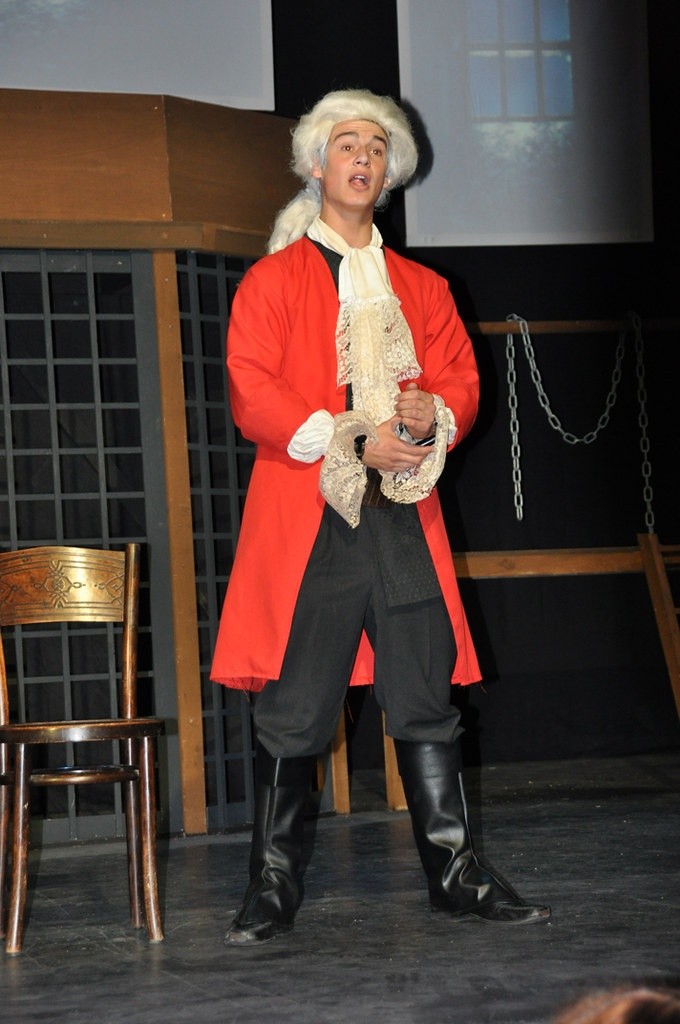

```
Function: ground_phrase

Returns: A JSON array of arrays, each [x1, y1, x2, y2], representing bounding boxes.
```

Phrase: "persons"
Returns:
[[210, 89, 555, 944]]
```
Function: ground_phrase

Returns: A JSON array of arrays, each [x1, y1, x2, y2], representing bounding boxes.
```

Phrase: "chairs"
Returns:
[[0, 540, 168, 958]]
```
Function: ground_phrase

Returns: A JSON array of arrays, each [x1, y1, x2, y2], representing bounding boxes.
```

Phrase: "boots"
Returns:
[[223, 735, 334, 945], [395, 733, 554, 926]]
